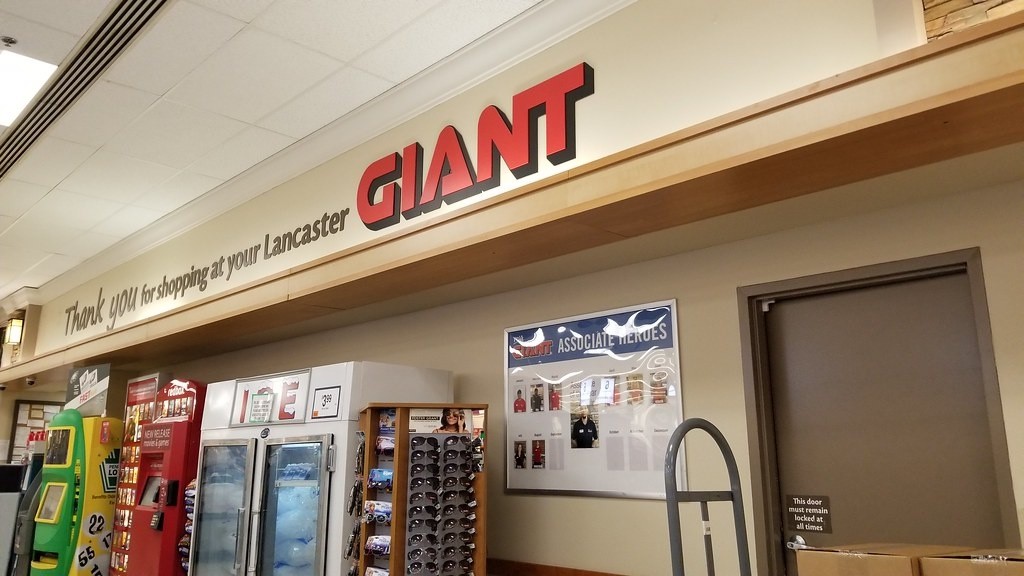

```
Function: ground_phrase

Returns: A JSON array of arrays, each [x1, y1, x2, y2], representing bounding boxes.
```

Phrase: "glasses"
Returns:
[[344, 433, 476, 576], [445, 411, 460, 417]]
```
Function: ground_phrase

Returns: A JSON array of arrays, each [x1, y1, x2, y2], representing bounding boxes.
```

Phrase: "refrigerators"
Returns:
[[187, 360, 454, 576]]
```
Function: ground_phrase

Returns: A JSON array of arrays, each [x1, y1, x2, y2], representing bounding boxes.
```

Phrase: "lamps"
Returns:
[[4, 317, 23, 345]]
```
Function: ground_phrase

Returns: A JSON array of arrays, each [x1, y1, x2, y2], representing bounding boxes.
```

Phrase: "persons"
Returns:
[[432, 409, 469, 433], [532, 441, 542, 468], [573, 405, 598, 448], [531, 388, 541, 412], [514, 443, 526, 468], [550, 384, 560, 410], [514, 389, 526, 412]]
